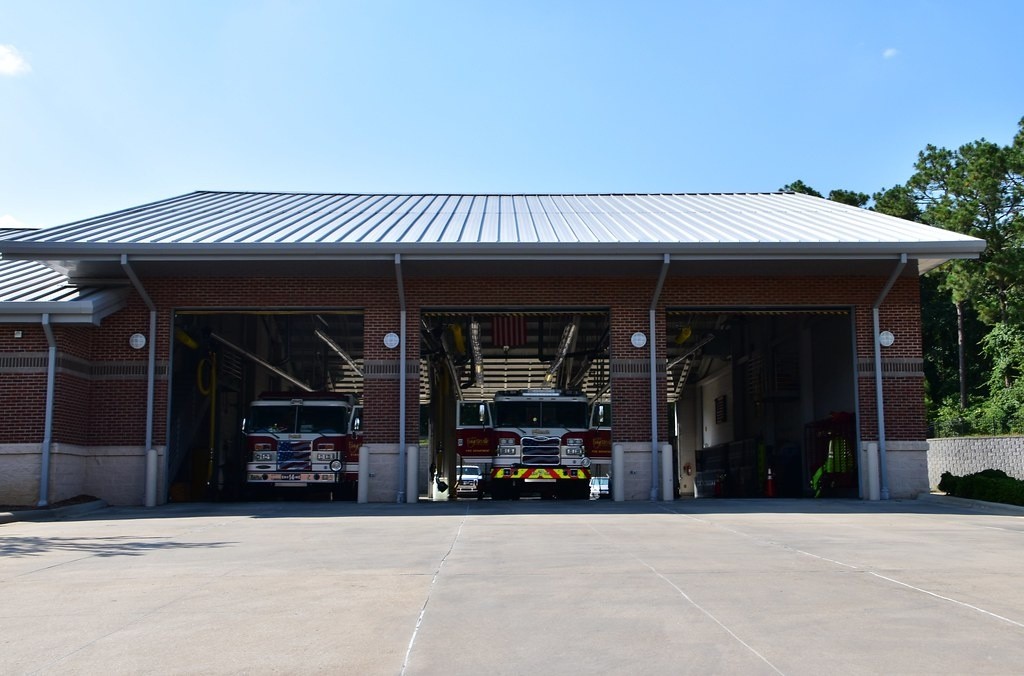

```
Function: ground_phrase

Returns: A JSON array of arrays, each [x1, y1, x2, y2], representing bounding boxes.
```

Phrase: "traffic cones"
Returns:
[[763, 467, 776, 498]]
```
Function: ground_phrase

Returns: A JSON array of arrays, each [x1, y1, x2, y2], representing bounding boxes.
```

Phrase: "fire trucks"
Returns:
[[239, 391, 362, 500], [455, 386, 611, 498]]
